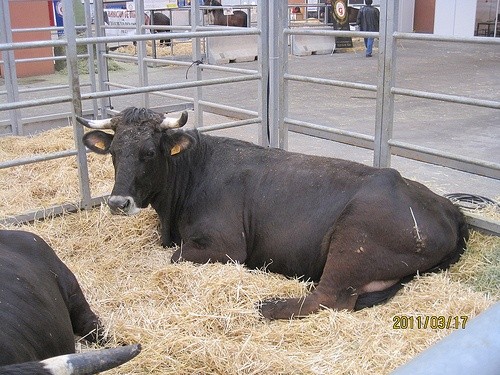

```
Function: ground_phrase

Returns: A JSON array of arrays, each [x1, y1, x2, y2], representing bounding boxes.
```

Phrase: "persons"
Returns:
[[356, 0, 380, 58]]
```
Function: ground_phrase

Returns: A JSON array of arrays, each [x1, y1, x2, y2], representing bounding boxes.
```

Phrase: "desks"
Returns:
[[477, 23, 500, 37]]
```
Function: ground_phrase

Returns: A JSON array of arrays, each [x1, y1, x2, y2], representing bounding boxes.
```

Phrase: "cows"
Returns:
[[0, 230, 141, 375], [74, 107, 469, 322]]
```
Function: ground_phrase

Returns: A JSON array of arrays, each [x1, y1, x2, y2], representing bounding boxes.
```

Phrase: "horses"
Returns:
[[149, 13, 170, 46], [203, 0, 247, 28]]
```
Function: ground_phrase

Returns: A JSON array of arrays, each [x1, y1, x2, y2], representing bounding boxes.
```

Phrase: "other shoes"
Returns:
[[366, 55, 373, 57]]
